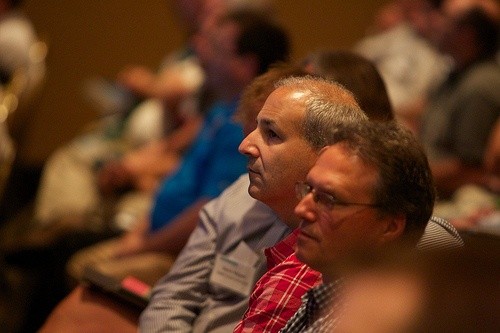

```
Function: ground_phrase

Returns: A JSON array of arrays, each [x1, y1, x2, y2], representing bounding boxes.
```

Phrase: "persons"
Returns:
[[2, 0, 499, 332]]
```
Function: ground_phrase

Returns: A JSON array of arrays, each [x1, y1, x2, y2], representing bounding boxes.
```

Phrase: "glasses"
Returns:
[[294, 181, 388, 210]]
